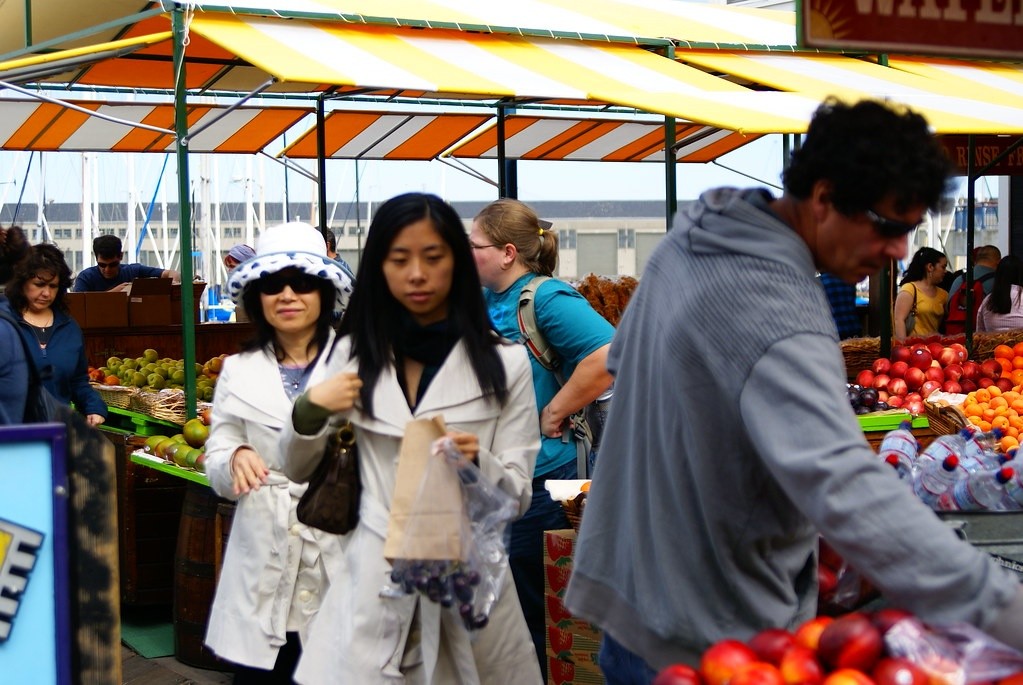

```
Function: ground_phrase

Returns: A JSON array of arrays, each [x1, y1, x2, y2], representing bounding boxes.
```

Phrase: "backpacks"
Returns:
[[944, 271, 996, 336]]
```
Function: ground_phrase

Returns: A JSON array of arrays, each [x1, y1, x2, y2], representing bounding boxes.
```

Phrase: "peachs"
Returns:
[[952, 384, 1023, 454], [653, 536, 1023, 685], [856, 340, 1013, 414]]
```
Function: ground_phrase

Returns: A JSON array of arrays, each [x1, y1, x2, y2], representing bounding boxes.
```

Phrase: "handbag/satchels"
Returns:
[[904, 282, 917, 337], [296, 423, 363, 536], [379, 441, 520, 630]]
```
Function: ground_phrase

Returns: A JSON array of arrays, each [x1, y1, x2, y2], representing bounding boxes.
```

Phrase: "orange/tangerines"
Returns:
[[993, 341, 1023, 385]]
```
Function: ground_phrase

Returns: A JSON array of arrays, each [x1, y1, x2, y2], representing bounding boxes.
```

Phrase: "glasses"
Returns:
[[225, 264, 235, 269], [253, 271, 327, 295], [861, 208, 922, 242], [97, 261, 120, 269]]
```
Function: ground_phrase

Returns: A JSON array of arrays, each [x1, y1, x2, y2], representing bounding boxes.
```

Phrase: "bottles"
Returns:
[[879, 419, 1023, 512]]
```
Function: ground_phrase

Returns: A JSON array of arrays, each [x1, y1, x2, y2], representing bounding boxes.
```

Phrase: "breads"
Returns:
[[576, 272, 639, 328]]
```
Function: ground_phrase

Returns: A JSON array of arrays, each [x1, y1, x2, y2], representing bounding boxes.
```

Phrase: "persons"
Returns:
[[468, 197, 622, 685], [569, 93, 1023, 685], [813, 245, 1023, 337], [224, 244, 257, 272], [314, 226, 354, 277], [0, 226, 108, 430], [74, 235, 201, 293], [202, 223, 358, 685], [275, 194, 549, 685]]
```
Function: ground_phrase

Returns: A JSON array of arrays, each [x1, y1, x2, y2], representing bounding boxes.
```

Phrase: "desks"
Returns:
[[95, 430, 216, 623]]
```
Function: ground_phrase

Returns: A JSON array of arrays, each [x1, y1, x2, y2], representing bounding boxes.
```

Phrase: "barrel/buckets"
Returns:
[[173, 487, 234, 673]]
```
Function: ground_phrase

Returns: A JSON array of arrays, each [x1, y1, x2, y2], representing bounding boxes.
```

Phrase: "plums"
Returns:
[[848, 384, 897, 415]]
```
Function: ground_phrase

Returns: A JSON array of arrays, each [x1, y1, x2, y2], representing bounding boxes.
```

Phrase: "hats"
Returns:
[[227, 220, 357, 321], [229, 244, 257, 262]]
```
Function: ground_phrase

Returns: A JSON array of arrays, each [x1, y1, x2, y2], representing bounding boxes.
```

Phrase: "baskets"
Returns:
[[839, 327, 1023, 455], [559, 498, 587, 536], [91, 384, 139, 410], [129, 391, 211, 427]]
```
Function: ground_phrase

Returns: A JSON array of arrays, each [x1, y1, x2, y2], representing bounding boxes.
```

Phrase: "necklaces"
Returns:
[[278, 361, 305, 389], [33, 308, 53, 332]]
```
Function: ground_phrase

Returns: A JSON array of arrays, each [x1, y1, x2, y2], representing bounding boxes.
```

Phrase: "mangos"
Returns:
[[142, 408, 213, 474]]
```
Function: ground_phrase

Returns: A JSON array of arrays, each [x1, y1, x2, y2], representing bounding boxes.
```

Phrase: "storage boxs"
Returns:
[[542, 528, 607, 685], [66, 277, 208, 327]]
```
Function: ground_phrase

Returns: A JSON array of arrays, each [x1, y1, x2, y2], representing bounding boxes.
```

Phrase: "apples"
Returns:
[[88, 348, 231, 402]]
